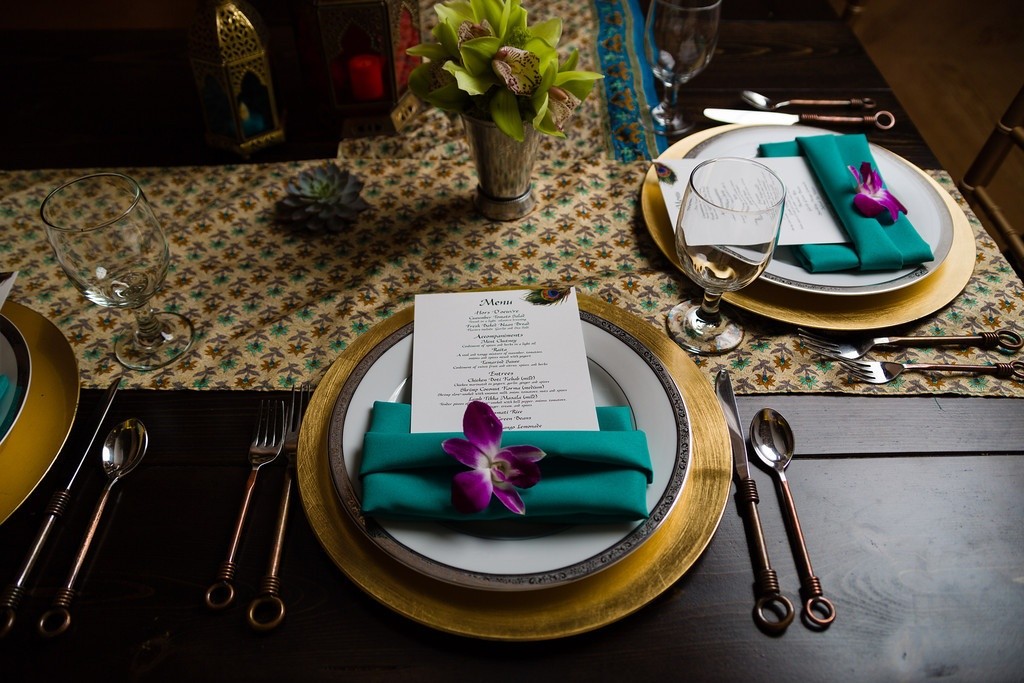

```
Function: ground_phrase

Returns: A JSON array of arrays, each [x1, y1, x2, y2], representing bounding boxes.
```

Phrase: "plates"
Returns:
[[296, 283, 734, 642], [641, 124, 976, 330], [0, 300, 81, 526]]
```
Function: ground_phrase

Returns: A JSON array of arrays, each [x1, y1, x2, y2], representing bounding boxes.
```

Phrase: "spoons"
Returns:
[[38, 418, 150, 637], [738, 89, 878, 112], [749, 407, 836, 627]]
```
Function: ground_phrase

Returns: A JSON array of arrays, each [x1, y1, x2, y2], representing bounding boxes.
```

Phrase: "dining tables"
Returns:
[[0, 0, 1024, 683]]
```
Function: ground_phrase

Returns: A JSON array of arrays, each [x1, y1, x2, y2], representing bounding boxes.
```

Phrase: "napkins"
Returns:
[[759, 134, 935, 274], [360, 401, 654, 521]]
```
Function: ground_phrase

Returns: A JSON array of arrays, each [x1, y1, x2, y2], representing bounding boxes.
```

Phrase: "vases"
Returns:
[[461, 111, 541, 220]]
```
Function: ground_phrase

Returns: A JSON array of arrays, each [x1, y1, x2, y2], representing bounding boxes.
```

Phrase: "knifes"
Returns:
[[0, 375, 121, 636], [703, 108, 897, 129], [715, 367, 794, 631]]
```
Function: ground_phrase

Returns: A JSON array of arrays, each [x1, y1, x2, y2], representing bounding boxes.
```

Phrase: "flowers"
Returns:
[[847, 162, 908, 221], [443, 401, 547, 516], [407, 0, 605, 141]]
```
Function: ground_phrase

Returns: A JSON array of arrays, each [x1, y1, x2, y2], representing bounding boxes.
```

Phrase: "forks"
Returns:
[[206, 382, 311, 629], [794, 324, 1024, 384]]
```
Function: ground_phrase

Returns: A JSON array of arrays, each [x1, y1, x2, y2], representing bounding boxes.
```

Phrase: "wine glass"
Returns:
[[38, 172, 195, 372], [637, 0, 721, 134], [662, 157, 788, 355]]
